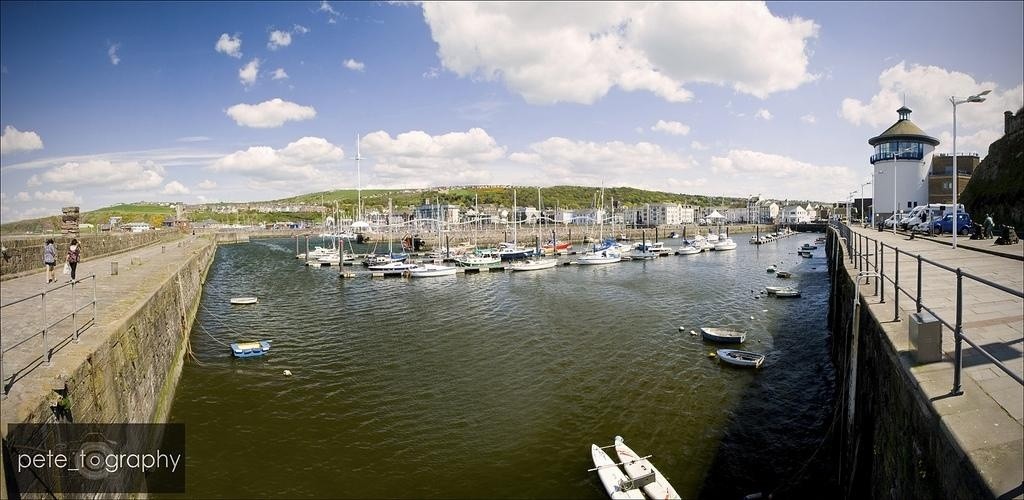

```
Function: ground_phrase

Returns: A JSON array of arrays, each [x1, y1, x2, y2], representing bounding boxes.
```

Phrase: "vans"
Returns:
[[884, 203, 972, 235]]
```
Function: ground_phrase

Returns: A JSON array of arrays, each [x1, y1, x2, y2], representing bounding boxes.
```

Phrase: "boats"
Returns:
[[798, 237, 826, 258], [700, 327, 747, 344], [776, 272, 791, 278], [766, 266, 777, 272], [613, 435, 681, 499], [765, 286, 802, 294], [716, 348, 765, 369], [749, 227, 792, 244], [587, 436, 646, 500], [776, 290, 798, 296], [229, 297, 258, 305], [230, 342, 270, 358]]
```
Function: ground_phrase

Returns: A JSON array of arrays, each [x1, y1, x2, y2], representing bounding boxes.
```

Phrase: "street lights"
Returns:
[[846, 190, 857, 227], [872, 170, 888, 230], [892, 146, 918, 234], [946, 89, 991, 249], [860, 181, 872, 229]]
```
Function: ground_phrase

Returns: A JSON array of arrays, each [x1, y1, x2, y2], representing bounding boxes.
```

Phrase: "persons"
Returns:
[[67, 239, 81, 283], [983, 213, 995, 239], [44, 238, 57, 284]]
